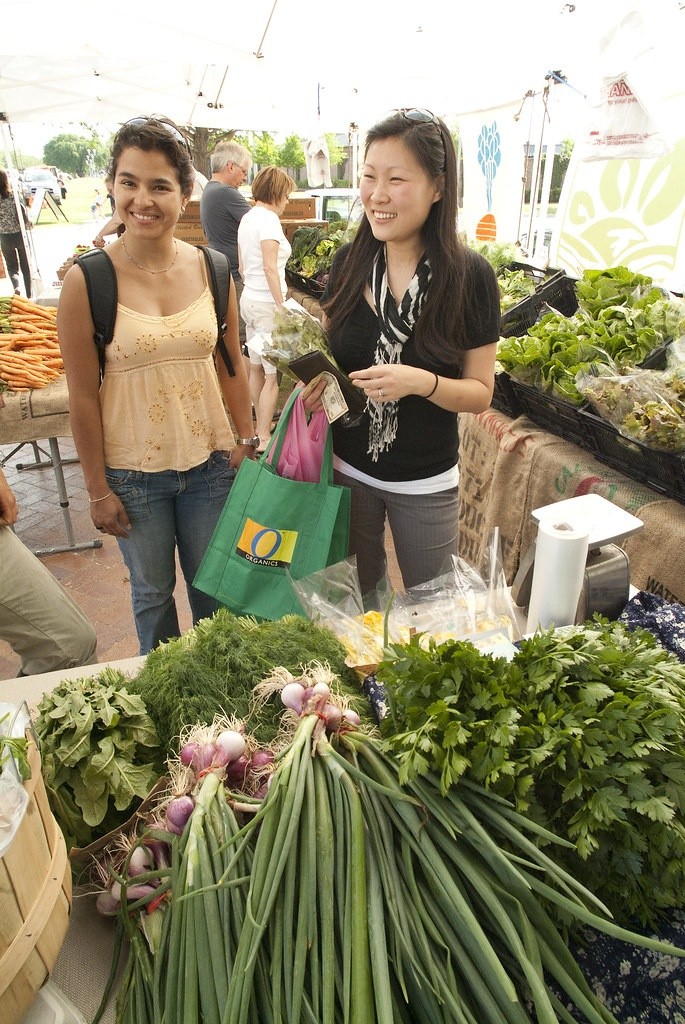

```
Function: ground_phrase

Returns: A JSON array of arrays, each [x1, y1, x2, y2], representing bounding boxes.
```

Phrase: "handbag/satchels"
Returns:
[[192, 387, 351, 623], [0, 251, 6, 278]]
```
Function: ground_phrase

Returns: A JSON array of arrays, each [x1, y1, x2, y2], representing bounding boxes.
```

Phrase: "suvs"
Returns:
[[21, 169, 62, 205]]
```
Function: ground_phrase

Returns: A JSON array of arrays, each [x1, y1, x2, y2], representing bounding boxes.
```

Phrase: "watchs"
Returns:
[[236, 437, 260, 448]]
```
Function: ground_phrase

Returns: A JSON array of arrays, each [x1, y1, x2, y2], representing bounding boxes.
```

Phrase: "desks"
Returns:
[[288, 283, 685, 612], [0, 369, 101, 557], [0, 572, 644, 1023]]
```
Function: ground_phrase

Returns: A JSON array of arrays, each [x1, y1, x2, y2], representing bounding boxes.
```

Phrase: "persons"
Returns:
[[198, 141, 282, 422], [0, 463, 102, 678], [236, 164, 291, 457], [0, 172, 35, 300], [57, 115, 260, 658], [293, 107, 502, 616]]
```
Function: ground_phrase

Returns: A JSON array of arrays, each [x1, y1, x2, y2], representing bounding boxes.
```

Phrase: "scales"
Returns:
[[511, 493, 644, 625]]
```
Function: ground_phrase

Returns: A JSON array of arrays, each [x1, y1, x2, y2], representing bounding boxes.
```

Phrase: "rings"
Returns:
[[378, 389, 383, 396]]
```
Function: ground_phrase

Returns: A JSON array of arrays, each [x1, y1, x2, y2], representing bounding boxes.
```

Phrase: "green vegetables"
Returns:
[[259, 316, 341, 383], [291, 219, 361, 276], [466, 240, 685, 455], [0, 607, 356, 872], [373, 611, 685, 931]]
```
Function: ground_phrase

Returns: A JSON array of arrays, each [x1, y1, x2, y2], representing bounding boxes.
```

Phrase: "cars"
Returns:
[[44, 165, 73, 182]]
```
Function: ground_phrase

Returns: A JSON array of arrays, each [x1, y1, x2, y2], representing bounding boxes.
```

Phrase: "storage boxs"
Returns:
[[284, 246, 685, 507], [173, 197, 329, 252]]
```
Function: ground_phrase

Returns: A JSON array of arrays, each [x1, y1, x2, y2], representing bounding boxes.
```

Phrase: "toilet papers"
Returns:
[[525, 514, 590, 638]]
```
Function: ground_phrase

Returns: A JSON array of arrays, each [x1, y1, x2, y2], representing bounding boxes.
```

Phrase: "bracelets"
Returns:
[[89, 490, 114, 503], [423, 370, 438, 398]]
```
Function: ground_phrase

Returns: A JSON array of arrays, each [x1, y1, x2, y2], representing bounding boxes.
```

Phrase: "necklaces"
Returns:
[[120, 232, 179, 274]]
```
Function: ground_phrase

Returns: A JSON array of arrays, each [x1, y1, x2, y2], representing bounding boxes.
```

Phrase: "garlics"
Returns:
[[96, 684, 361, 915]]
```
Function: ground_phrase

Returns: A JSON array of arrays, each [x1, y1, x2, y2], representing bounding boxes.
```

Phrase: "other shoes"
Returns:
[[15, 289, 21, 296]]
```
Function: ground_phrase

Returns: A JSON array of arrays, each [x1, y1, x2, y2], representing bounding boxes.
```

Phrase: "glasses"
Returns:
[[284, 192, 289, 200], [227, 161, 248, 176], [376, 107, 441, 134], [117, 118, 187, 154]]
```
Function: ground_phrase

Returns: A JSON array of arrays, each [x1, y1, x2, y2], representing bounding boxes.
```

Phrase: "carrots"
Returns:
[[0, 295, 65, 391]]
